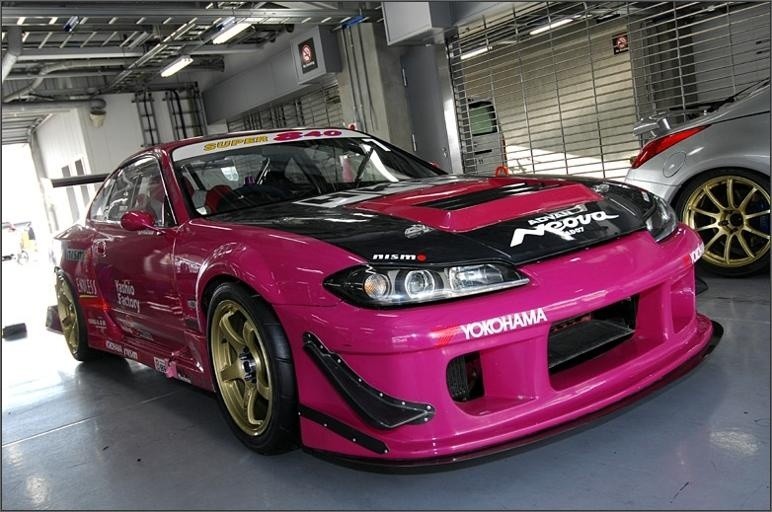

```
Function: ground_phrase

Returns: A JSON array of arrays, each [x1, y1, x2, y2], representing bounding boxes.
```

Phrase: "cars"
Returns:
[[2, 223, 37, 263]]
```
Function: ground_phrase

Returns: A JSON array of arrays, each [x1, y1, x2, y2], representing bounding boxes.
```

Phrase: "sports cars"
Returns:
[[619, 73, 771, 275], [42, 122, 729, 471]]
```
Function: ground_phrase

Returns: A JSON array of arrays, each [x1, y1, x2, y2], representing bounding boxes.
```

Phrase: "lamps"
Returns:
[[159, 54, 194, 77]]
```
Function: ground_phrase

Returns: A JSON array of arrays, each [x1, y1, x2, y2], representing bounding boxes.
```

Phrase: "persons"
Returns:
[[19, 227, 32, 251], [26, 222, 39, 263]]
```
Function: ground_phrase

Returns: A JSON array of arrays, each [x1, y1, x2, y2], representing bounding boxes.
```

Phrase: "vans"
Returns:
[[468, 100, 506, 176]]
